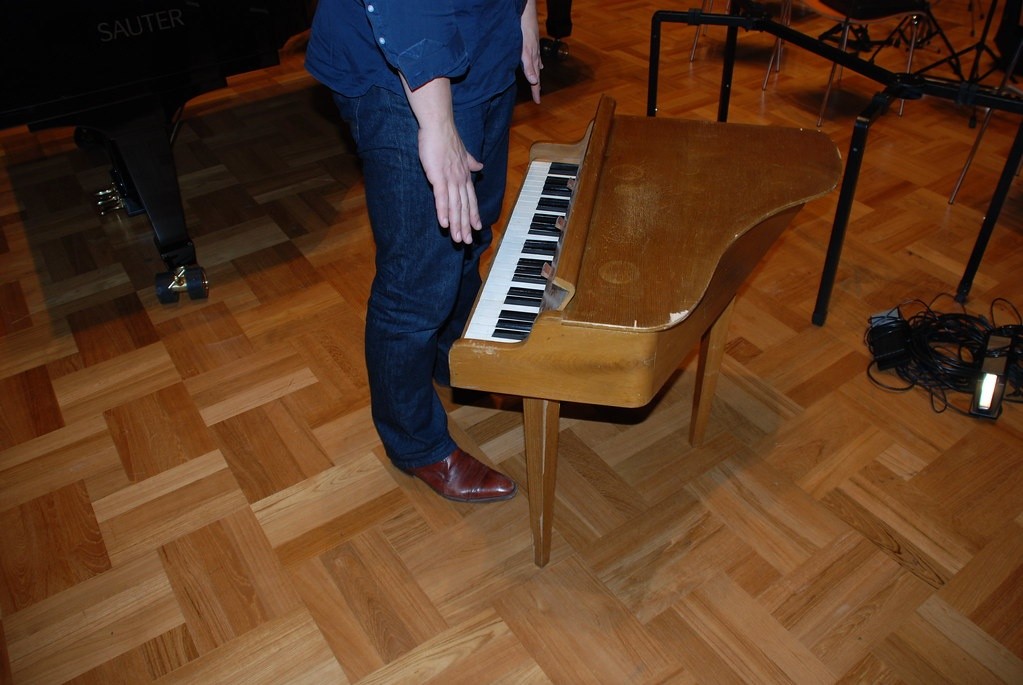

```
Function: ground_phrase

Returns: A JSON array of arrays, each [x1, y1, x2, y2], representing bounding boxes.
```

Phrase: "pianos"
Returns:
[[448, 94, 844, 569]]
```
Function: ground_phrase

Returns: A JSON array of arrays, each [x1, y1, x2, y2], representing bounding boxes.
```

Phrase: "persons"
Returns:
[[304, 0, 545, 502]]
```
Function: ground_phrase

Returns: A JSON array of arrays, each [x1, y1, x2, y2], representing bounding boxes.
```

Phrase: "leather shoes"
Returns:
[[391, 446, 518, 503]]
